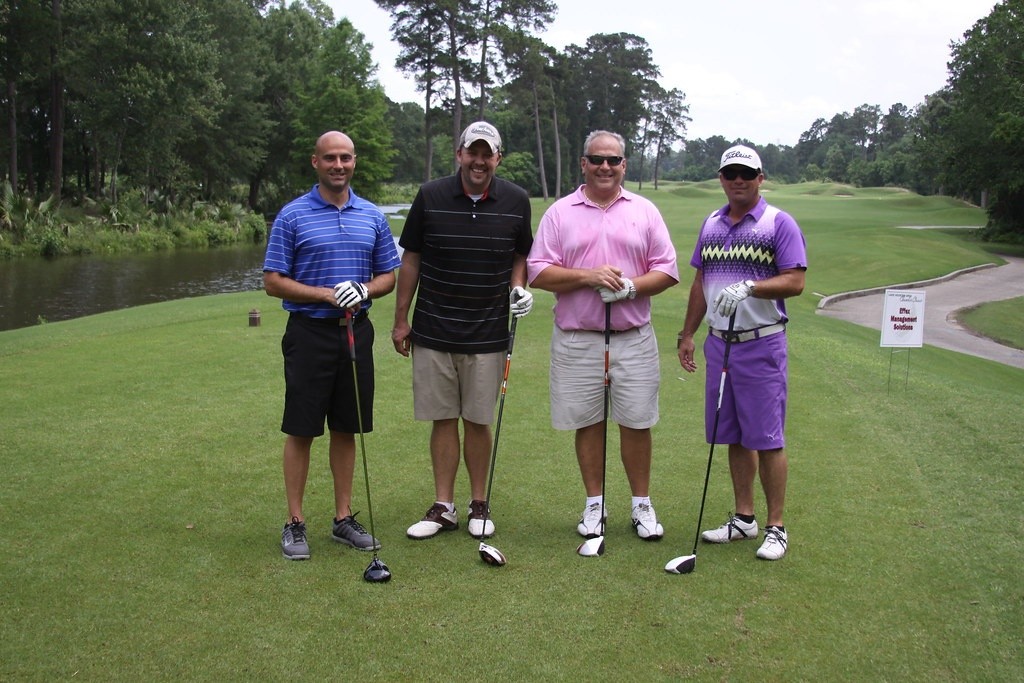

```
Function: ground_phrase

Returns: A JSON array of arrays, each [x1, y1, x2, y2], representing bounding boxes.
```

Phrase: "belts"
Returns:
[[296, 311, 367, 326], [589, 327, 635, 334], [709, 323, 785, 342]]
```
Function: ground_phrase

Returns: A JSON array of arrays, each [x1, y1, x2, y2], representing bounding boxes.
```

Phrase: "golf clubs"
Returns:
[[575, 302, 613, 558], [477, 293, 523, 567], [345, 308, 392, 584], [664, 305, 740, 576]]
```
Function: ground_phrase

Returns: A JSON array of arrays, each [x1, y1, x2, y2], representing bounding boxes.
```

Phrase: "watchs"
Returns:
[[745, 281, 755, 293], [628, 280, 636, 300]]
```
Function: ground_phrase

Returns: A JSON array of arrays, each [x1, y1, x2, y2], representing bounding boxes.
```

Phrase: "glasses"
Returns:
[[585, 155, 623, 166], [720, 168, 758, 181]]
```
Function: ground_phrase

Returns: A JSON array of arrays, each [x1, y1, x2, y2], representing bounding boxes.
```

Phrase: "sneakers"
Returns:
[[756, 525, 787, 560], [577, 502, 608, 536], [406, 501, 458, 538], [282, 522, 311, 560], [332, 517, 382, 551], [631, 502, 664, 540], [702, 517, 758, 542], [468, 501, 495, 537]]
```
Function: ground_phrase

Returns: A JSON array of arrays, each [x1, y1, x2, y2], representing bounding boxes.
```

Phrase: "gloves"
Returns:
[[713, 283, 751, 317], [334, 280, 368, 308], [593, 278, 636, 302], [510, 287, 533, 317]]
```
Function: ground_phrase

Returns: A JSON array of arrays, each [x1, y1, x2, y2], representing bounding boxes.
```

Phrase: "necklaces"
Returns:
[[583, 189, 620, 208]]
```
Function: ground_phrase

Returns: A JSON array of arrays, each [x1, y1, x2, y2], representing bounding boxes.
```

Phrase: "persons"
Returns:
[[392, 121, 534, 540], [526, 130, 679, 539], [263, 130, 400, 560], [676, 144, 807, 559]]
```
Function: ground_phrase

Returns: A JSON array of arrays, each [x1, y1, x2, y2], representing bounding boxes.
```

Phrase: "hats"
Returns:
[[459, 121, 501, 153], [717, 146, 762, 172]]
